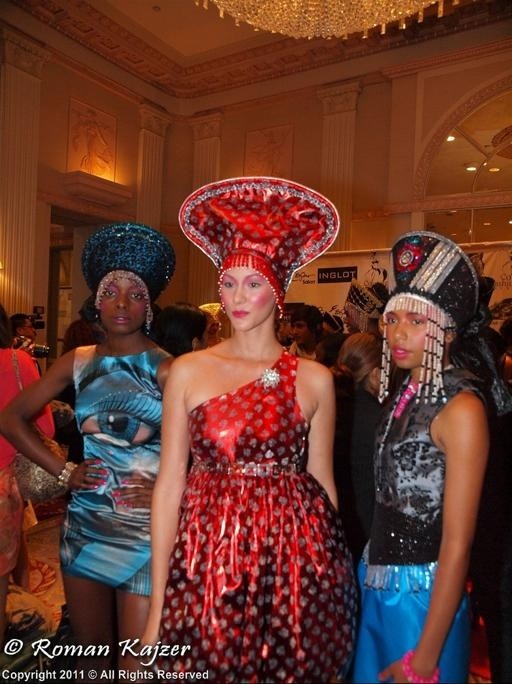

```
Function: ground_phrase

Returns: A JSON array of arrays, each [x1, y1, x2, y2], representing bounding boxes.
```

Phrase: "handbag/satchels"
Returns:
[[12, 439, 68, 500]]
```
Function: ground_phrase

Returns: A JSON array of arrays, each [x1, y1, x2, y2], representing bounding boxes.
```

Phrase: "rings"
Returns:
[[23, 343, 28, 347], [31, 350, 35, 355]]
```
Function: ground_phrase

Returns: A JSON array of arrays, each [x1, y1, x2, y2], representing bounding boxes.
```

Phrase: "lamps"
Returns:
[[189, 0, 460, 41]]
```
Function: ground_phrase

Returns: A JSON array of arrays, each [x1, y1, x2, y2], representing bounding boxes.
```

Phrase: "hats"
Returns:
[[378, 230, 479, 403], [81, 223, 176, 330], [178, 176, 340, 320], [343, 278, 388, 330]]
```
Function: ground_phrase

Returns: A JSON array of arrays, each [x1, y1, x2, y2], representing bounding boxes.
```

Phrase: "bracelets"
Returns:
[[402, 651, 441, 683]]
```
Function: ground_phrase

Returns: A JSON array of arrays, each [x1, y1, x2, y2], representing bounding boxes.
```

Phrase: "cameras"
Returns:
[[15, 335, 50, 358]]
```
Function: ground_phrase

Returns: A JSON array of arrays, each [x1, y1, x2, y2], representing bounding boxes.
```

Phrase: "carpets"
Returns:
[[7, 494, 72, 633]]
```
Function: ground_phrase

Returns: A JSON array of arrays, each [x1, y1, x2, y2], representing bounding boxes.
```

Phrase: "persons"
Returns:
[[56, 292, 511, 575], [10, 312, 37, 343], [355, 293, 491, 683], [139, 252, 360, 683], [1, 270, 179, 682], [0, 305, 56, 650]]
